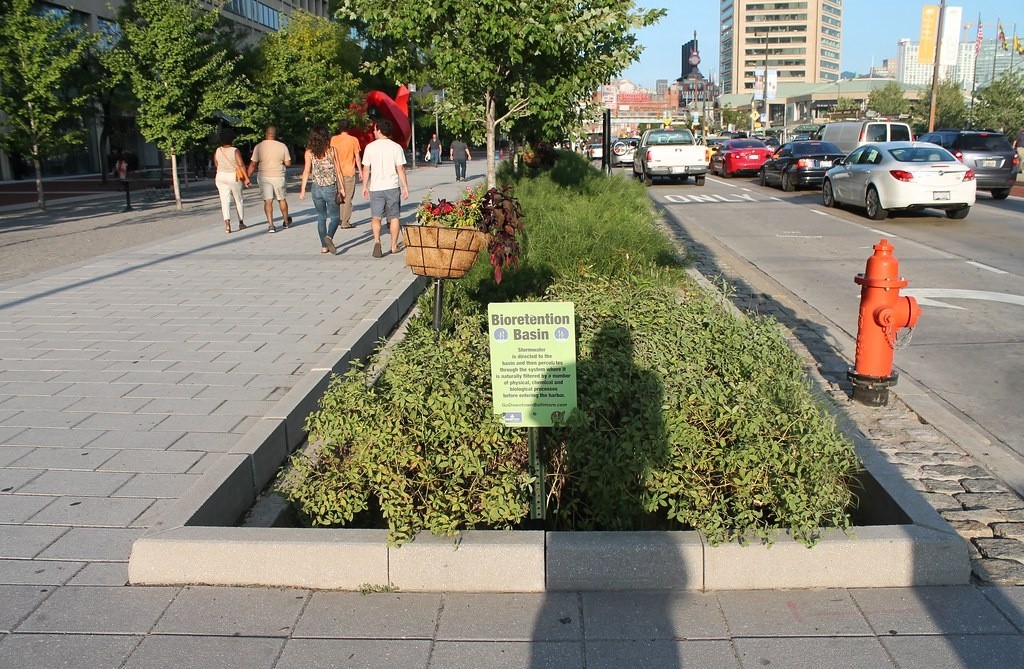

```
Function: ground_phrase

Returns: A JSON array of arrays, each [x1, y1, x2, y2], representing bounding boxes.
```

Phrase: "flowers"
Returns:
[[415, 185, 525, 284]]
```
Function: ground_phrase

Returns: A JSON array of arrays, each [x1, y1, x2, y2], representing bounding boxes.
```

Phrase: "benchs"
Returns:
[[896, 153, 941, 162]]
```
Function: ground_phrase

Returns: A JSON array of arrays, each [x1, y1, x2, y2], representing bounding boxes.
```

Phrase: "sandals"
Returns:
[[341, 225, 354, 229]]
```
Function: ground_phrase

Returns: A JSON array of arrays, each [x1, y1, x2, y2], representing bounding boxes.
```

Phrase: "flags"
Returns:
[[974, 20, 983, 56], [1014, 33, 1024, 59], [998, 24, 1009, 50]]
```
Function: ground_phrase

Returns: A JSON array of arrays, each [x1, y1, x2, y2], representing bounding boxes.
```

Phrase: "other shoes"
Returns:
[[268, 227, 276, 232], [226, 226, 231, 233], [282, 217, 293, 228], [324, 236, 336, 254], [457, 178, 464, 182], [239, 224, 245, 229], [322, 248, 328, 253]]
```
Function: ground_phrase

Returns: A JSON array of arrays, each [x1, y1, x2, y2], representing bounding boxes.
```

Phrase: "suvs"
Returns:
[[915, 129, 1019, 199]]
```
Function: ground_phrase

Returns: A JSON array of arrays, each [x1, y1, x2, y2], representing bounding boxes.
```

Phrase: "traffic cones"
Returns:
[[500, 149, 505, 158]]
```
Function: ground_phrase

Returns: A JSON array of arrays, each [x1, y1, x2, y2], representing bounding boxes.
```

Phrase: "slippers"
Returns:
[[373, 244, 382, 258], [393, 246, 403, 254]]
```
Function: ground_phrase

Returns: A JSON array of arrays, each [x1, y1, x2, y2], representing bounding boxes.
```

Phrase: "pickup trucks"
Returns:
[[631, 128, 709, 187]]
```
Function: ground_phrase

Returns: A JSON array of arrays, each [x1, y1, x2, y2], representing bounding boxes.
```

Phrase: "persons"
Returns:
[[246, 125, 292, 232], [330, 119, 363, 229], [300, 122, 346, 254], [361, 121, 409, 258], [214, 129, 251, 234], [450, 132, 472, 182], [427, 133, 442, 167], [565, 139, 592, 161]]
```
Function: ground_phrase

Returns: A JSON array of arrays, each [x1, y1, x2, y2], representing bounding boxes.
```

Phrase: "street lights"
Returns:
[[408, 83, 416, 166], [433, 94, 441, 163]]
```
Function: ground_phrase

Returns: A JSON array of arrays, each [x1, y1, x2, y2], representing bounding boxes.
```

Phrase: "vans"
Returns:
[[808, 120, 915, 162]]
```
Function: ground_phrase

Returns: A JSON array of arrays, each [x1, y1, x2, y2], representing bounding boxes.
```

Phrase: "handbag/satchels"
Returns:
[[235, 170, 245, 180], [336, 192, 345, 205], [425, 150, 431, 161]]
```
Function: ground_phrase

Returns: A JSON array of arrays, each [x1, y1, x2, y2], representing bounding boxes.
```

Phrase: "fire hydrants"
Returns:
[[847, 237, 923, 407]]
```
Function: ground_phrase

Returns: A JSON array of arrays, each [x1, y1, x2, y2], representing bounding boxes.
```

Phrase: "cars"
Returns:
[[788, 124, 814, 142], [710, 139, 773, 178], [760, 142, 848, 192], [755, 136, 781, 151], [697, 137, 732, 163], [823, 141, 977, 220], [587, 144, 603, 160], [718, 131, 747, 138], [610, 137, 641, 167]]
